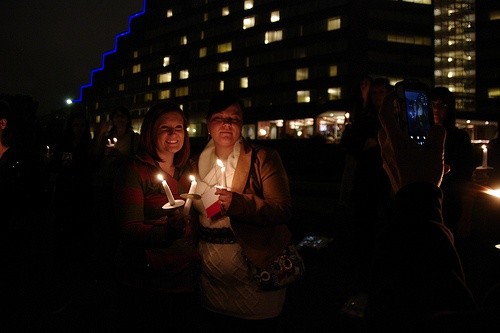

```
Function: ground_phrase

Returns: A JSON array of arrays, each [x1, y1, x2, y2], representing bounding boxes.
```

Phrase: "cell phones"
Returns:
[[403, 80, 436, 148]]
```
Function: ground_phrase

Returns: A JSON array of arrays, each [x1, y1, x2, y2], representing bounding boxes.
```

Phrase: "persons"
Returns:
[[278, 86, 499, 333], [0, 94, 145, 333], [116, 102, 201, 333], [191, 98, 292, 333]]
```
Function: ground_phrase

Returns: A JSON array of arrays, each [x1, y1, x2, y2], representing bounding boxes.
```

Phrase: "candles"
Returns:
[[215, 158, 226, 189], [108, 139, 111, 145], [156, 173, 175, 206], [481, 144, 488, 167], [183, 174, 198, 216], [46, 145, 50, 158], [113, 137, 118, 144]]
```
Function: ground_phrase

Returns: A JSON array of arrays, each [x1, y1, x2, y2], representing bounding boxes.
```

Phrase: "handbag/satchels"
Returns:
[[252, 249, 305, 292]]
